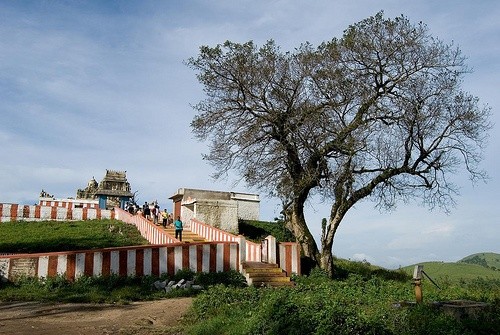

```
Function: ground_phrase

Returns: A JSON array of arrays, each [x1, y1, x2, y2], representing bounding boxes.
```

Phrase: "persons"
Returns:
[[174, 216, 184, 241], [152, 205, 160, 225], [143, 202, 149, 212], [161, 209, 169, 229], [144, 205, 150, 220], [124, 201, 141, 215]]
